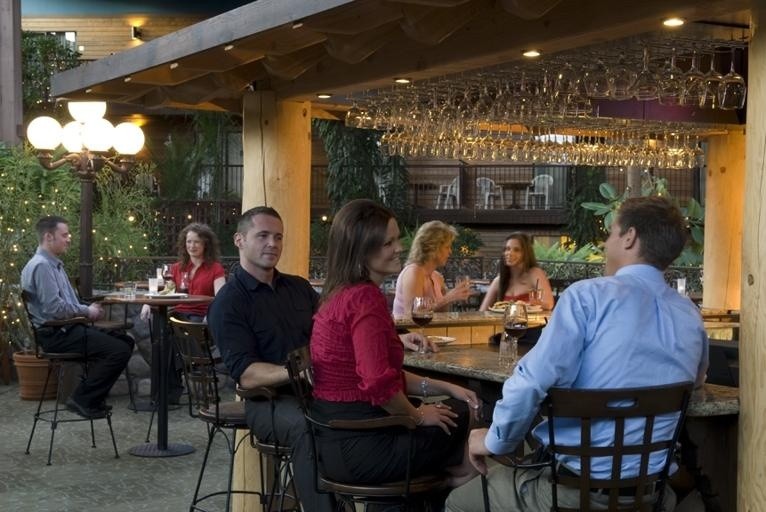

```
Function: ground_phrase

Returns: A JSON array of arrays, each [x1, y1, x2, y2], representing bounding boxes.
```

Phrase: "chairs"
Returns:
[[232, 383, 304, 511], [479, 382, 698, 511], [476, 177, 504, 210], [306, 413, 446, 511], [17, 288, 119, 465], [170, 315, 266, 511], [435, 174, 461, 209], [524, 174, 554, 210]]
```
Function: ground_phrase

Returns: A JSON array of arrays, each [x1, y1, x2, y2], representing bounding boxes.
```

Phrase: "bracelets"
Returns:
[[420, 377, 431, 397], [416, 406, 424, 426]]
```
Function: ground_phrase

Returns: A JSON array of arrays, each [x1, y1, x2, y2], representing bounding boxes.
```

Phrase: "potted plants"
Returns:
[[0, 140, 149, 400]]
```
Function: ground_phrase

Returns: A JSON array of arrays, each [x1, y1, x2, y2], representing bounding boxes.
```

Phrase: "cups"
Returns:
[[180, 272, 189, 293], [411, 296, 433, 357], [149, 278, 158, 295], [499, 332, 515, 370], [504, 304, 528, 368], [161, 264, 174, 282], [456, 276, 469, 287], [123, 282, 137, 299]]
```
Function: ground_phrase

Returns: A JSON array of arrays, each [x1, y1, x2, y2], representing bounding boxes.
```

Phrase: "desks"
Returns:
[[398, 307, 553, 343], [401, 341, 739, 418], [105, 292, 214, 457], [408, 182, 438, 209], [116, 279, 164, 325], [699, 302, 740, 339], [497, 181, 529, 209]]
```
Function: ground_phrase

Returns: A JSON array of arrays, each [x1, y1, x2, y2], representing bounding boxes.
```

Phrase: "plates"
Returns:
[[488, 305, 543, 313], [427, 335, 456, 346], [144, 292, 188, 298]]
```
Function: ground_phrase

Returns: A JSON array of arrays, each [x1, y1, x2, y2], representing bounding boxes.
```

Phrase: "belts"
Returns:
[[557, 462, 661, 498]]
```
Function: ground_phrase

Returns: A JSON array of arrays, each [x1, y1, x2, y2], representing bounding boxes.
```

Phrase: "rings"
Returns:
[[466, 399, 471, 402]]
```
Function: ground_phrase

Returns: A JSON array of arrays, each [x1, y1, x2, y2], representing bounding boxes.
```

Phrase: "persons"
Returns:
[[208, 207, 337, 511], [445, 194, 704, 512], [310, 199, 484, 512], [479, 232, 554, 312], [21, 216, 135, 419], [141, 222, 227, 405], [393, 221, 471, 320]]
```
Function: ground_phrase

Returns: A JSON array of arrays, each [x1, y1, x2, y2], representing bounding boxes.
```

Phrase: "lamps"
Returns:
[[26, 101, 146, 296], [395, 77, 410, 84], [317, 93, 333, 99], [661, 16, 688, 28], [131, 26, 142, 40], [521, 49, 542, 57]]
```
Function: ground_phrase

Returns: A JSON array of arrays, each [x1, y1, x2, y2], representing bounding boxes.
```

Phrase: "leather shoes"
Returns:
[[64, 397, 113, 419]]
